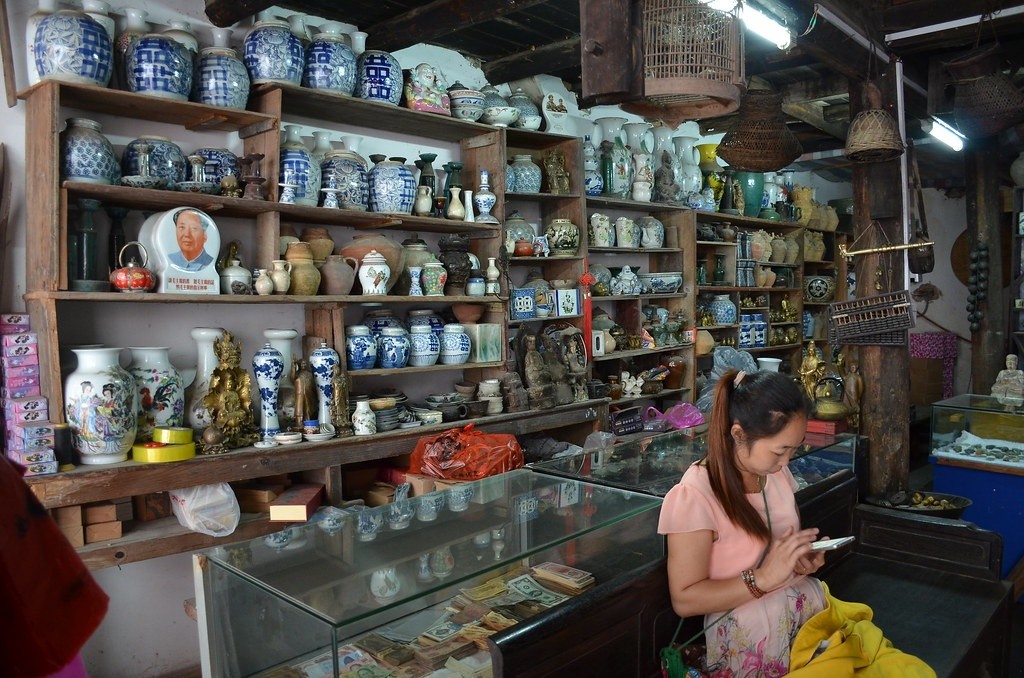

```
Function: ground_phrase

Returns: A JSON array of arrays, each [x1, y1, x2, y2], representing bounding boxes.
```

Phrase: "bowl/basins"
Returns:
[[862, 491, 973, 521]]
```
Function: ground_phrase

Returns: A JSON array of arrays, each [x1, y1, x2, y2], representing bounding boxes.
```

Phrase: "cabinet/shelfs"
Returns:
[[16, 80, 853, 512]]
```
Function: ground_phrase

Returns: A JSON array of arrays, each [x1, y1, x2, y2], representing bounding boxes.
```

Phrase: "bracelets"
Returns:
[[741, 569, 767, 599]]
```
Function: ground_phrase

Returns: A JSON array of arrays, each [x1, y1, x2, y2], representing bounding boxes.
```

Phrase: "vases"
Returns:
[[59, 342, 106, 382], [62, 348, 137, 464], [124, 346, 184, 446], [261, 326, 297, 428], [185, 326, 227, 434]]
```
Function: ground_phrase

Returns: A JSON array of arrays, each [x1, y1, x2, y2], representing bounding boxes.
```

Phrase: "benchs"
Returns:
[[486, 473, 1013, 678]]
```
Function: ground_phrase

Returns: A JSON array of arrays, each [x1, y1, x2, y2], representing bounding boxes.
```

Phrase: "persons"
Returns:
[[797, 340, 824, 402], [291, 358, 316, 427], [610, 265, 643, 295], [991, 354, 1024, 398], [405, 62, 451, 115], [167, 208, 214, 271], [836, 353, 864, 426], [657, 369, 829, 678], [525, 337, 551, 388], [219, 372, 246, 428], [1, 456, 110, 678], [503, 359, 526, 408], [770, 326, 798, 346], [332, 365, 350, 426]]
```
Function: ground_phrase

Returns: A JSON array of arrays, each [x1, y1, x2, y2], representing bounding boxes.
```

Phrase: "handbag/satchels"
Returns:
[[660, 648, 703, 678]]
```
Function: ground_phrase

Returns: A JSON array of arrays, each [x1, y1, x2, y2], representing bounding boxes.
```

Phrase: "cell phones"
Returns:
[[809, 536, 855, 552]]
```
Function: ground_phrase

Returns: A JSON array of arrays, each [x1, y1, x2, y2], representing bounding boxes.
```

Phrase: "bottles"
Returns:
[[24, 0, 817, 470], [260, 485, 504, 603]]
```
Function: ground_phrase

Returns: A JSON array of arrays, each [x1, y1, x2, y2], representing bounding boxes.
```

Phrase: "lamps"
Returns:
[[703, 0, 799, 50], [921, 115, 968, 152]]
